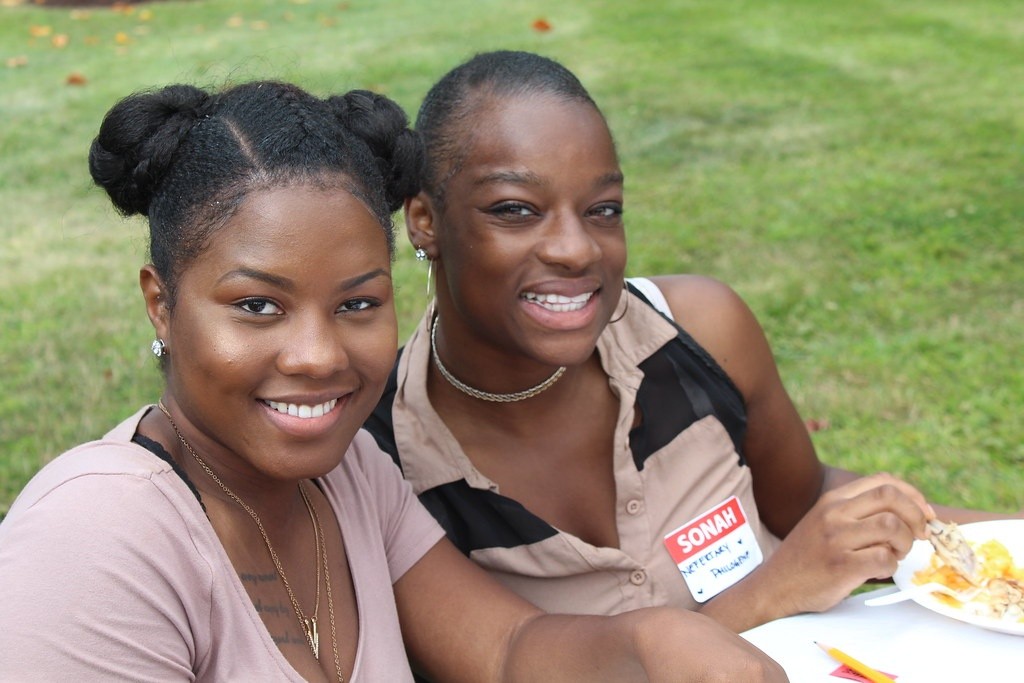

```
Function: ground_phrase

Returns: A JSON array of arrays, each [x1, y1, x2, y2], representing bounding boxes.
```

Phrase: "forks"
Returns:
[[864, 576, 990, 608]]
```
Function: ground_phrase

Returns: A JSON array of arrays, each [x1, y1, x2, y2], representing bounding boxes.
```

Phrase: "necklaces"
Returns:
[[154, 396, 344, 681], [432, 315, 568, 404]]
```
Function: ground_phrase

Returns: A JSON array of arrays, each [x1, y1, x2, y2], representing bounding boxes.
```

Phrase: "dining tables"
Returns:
[[738, 584, 1024, 683]]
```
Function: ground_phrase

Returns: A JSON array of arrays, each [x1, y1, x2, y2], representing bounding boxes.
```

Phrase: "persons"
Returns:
[[0, 82, 790, 682], [361, 53, 1023, 632]]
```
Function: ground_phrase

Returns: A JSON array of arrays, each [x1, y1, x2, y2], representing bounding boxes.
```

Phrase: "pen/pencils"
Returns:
[[813, 639, 896, 683]]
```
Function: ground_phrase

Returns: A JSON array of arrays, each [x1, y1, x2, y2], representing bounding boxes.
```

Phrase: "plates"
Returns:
[[891, 519, 1024, 637]]
